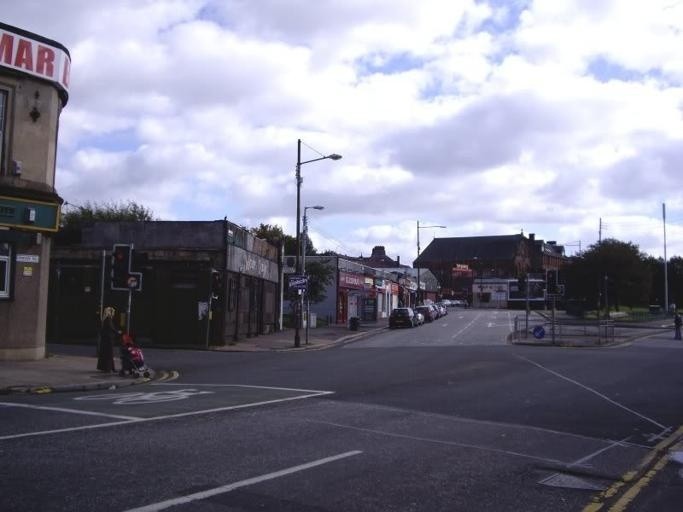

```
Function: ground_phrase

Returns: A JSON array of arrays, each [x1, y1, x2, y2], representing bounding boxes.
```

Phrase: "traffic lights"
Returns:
[[545, 268, 564, 297], [210, 269, 222, 298]]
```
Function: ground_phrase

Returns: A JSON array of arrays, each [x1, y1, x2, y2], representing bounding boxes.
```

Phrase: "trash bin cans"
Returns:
[[349, 316, 361, 331]]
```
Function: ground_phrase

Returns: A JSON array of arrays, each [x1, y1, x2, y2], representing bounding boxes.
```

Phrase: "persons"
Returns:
[[96, 307, 121, 374], [673, 311, 682, 340], [128, 342, 143, 371]]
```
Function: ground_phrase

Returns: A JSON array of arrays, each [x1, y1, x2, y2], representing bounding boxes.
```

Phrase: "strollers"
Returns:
[[119, 331, 149, 380]]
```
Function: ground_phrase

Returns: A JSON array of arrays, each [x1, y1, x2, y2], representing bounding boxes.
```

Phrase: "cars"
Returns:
[[413, 312, 423, 326], [432, 299, 460, 319]]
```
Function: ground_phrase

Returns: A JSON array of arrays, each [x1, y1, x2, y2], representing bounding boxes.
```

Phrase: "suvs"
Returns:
[[389, 307, 418, 327], [412, 305, 437, 323]]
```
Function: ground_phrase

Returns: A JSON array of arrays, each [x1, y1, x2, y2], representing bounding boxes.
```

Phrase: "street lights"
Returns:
[[416, 225, 446, 303], [296, 154, 340, 343]]
